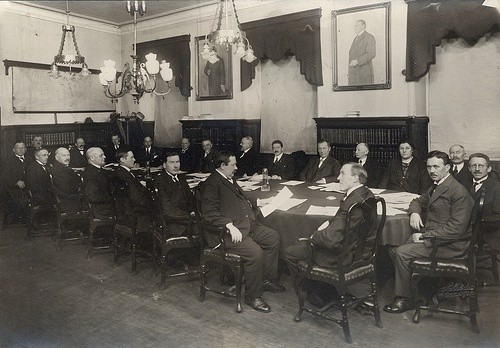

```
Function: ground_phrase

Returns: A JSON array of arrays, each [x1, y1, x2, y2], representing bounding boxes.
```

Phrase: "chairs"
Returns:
[[293, 195, 383, 343], [0, 169, 245, 313], [410, 189, 487, 334], [474, 170, 500, 284]]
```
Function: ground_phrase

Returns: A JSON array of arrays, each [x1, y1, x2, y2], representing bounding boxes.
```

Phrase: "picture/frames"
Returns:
[[331, 1, 392, 91], [193, 35, 232, 100]]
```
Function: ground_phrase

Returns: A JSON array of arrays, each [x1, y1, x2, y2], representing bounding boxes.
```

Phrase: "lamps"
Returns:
[[201, 0, 257, 64], [48, 0, 92, 81], [99, 0, 173, 103]]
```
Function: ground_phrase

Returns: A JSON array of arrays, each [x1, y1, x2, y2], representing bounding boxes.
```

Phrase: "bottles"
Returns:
[[262, 168, 268, 186]]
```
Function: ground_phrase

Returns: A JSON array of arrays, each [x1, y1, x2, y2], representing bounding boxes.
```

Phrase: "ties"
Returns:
[[318, 158, 324, 168], [454, 166, 458, 173], [116, 145, 119, 149], [429, 184, 437, 197], [130, 172, 135, 178], [20, 156, 25, 161], [240, 152, 245, 158], [275, 158, 278, 163], [474, 182, 480, 193], [174, 177, 187, 198], [147, 148, 149, 155], [229, 179, 235, 191]]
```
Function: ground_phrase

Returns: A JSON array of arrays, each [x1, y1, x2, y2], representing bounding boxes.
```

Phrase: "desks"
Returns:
[[71, 164, 419, 261]]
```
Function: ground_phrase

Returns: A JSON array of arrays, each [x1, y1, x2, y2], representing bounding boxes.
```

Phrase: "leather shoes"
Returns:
[[384, 297, 415, 314], [263, 279, 286, 293], [244, 296, 272, 313]]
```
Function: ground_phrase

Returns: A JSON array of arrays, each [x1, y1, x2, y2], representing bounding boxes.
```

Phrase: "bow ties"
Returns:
[[79, 149, 84, 151]]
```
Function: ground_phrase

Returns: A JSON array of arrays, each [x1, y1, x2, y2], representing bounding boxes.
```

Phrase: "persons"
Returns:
[[234, 136, 263, 175], [7, 135, 55, 205], [262, 140, 296, 179], [447, 144, 500, 244], [297, 138, 341, 183], [284, 162, 377, 312], [200, 150, 285, 313], [69, 138, 88, 166], [103, 131, 122, 163], [355, 142, 384, 187], [110, 147, 153, 224], [137, 136, 161, 164], [151, 150, 205, 272], [385, 150, 477, 313], [170, 136, 222, 173], [377, 138, 431, 195], [52, 146, 116, 248]]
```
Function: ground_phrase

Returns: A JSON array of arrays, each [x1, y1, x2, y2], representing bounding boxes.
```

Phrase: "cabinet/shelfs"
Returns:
[[315, 115, 430, 189], [0, 121, 155, 166], [178, 120, 261, 160]]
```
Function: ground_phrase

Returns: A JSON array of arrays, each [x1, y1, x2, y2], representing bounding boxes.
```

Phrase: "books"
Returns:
[[26, 132, 75, 147], [185, 126, 236, 142], [321, 128, 403, 167]]
